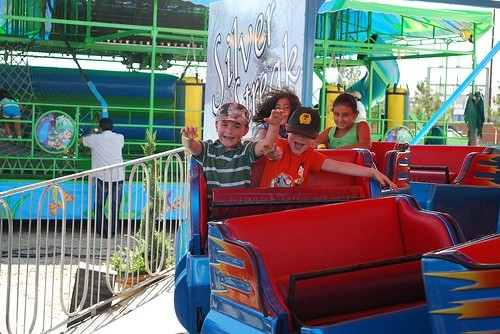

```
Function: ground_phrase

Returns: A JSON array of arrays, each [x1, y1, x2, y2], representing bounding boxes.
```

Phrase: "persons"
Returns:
[[180, 101, 287, 216], [252, 82, 302, 142], [0, 89, 25, 142], [313, 93, 371, 150], [261, 107, 400, 198], [353, 91, 367, 121], [80, 117, 125, 238]]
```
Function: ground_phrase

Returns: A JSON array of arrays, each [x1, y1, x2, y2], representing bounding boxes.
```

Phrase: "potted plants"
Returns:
[[107, 126, 176, 302]]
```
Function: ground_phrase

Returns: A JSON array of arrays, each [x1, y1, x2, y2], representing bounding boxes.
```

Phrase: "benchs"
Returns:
[[204, 194, 459, 331], [186, 147, 383, 254], [410, 145, 500, 203], [423, 235, 500, 333], [365, 141, 411, 194]]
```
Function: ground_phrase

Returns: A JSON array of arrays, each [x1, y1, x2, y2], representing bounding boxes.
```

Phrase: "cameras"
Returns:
[[93, 128, 99, 132]]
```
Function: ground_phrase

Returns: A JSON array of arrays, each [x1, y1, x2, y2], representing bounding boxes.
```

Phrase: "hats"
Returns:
[[286, 107, 320, 140], [215, 102, 251, 125]]
